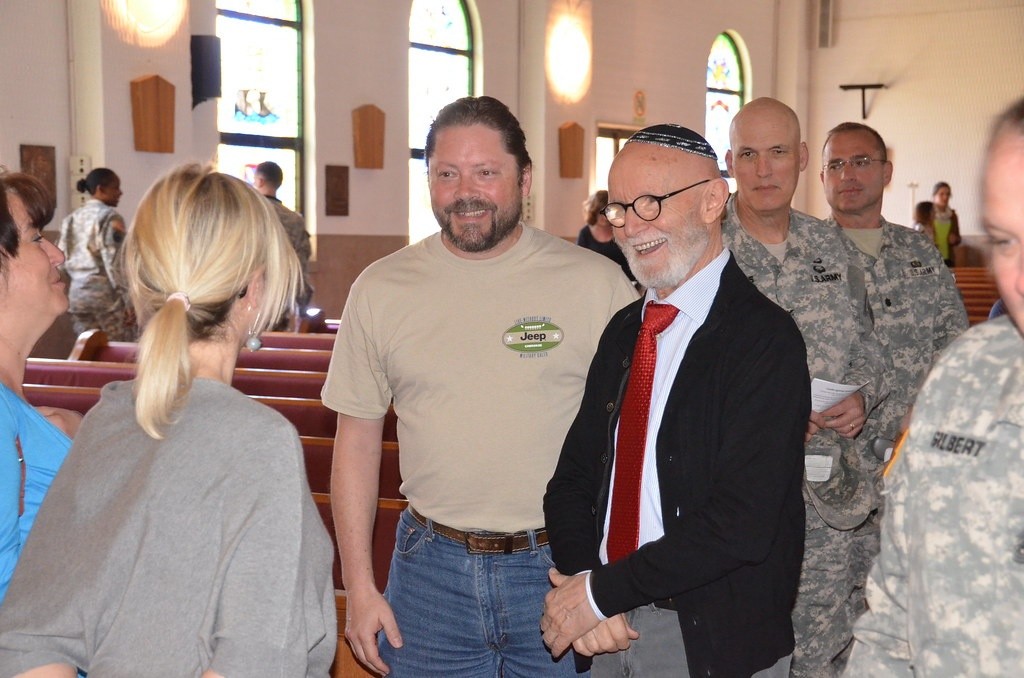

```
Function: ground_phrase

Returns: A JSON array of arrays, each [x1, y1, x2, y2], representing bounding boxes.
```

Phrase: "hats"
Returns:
[[803, 416, 872, 531], [624, 123, 719, 159]]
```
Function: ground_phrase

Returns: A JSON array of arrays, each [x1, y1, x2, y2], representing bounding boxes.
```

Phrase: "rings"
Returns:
[[850, 424, 854, 430]]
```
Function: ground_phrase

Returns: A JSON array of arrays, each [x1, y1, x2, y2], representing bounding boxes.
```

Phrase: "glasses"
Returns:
[[822, 156, 887, 172], [599, 179, 710, 228]]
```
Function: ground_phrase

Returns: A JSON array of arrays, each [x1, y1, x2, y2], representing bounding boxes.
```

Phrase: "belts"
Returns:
[[644, 597, 674, 611], [407, 503, 549, 555]]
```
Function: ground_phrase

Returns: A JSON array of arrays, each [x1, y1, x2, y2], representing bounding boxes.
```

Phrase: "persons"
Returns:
[[0, 168, 337, 678], [321, 96, 641, 678], [58, 169, 140, 343], [820, 122, 968, 609], [0, 173, 83, 604], [721, 98, 890, 678], [840, 96, 1024, 678], [541, 124, 813, 678], [933, 182, 961, 267], [576, 190, 641, 288], [253, 161, 311, 314], [914, 201, 937, 243]]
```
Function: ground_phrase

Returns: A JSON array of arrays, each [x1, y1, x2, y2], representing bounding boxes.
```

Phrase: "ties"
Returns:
[[607, 300, 680, 564]]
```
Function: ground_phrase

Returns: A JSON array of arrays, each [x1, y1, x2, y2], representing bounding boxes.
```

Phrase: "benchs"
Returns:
[[21, 320, 410, 594]]
